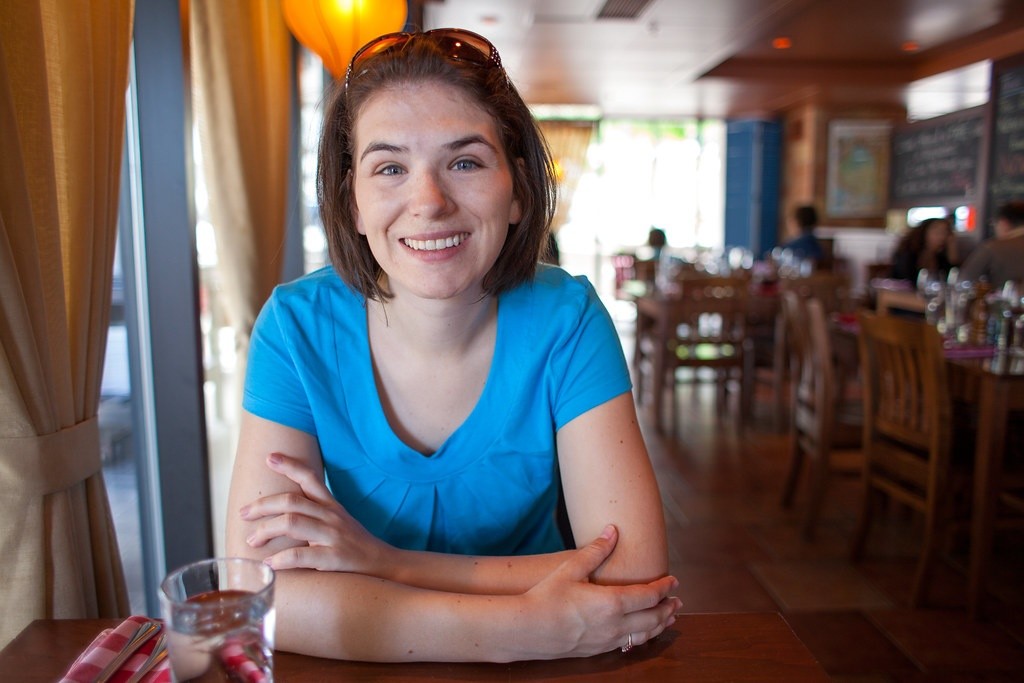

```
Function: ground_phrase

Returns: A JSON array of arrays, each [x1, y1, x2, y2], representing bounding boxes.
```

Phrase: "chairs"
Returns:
[[849, 308, 1024, 608], [667, 269, 744, 422], [775, 284, 868, 546]]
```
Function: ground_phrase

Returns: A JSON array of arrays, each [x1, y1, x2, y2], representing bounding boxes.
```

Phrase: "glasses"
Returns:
[[345, 27, 509, 119]]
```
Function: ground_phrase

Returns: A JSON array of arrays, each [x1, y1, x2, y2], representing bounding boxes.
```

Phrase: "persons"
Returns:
[[226, 29, 682, 663], [644, 230, 684, 262], [873, 200, 1024, 318], [774, 203, 823, 268]]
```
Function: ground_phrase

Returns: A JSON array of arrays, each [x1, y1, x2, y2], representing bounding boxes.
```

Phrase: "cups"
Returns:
[[156, 558, 276, 683]]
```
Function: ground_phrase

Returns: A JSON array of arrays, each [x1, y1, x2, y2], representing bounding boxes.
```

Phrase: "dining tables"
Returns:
[[635, 286, 774, 418], [937, 334, 1024, 623], [0, 608, 834, 683]]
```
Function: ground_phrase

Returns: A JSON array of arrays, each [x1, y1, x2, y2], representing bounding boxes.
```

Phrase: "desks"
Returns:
[[929, 329, 1023, 624]]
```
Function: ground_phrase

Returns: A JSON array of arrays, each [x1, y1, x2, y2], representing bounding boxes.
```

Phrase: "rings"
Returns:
[[622, 634, 632, 652]]
